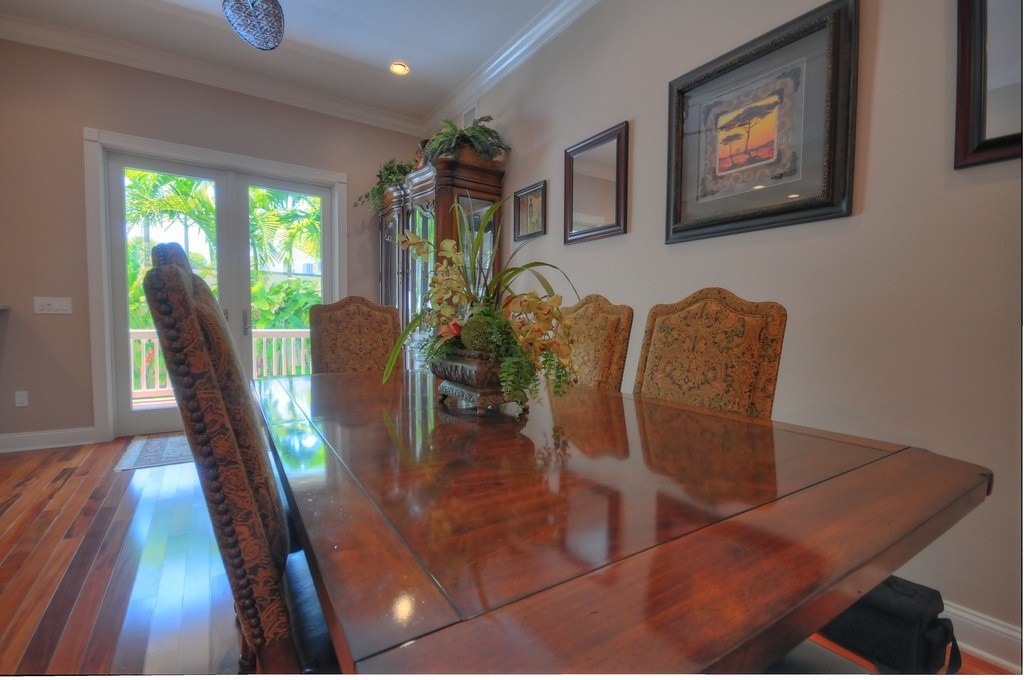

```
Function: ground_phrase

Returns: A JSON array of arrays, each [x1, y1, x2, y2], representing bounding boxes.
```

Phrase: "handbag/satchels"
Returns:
[[814, 575, 962, 674]]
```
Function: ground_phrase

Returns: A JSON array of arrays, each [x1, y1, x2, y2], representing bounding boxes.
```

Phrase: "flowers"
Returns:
[[378, 194, 584, 408]]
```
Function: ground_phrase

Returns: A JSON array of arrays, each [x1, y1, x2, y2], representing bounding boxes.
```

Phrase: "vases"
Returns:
[[430, 349, 530, 417]]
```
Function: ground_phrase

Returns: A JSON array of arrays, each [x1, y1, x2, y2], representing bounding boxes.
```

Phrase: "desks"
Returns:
[[258, 371, 991, 674]]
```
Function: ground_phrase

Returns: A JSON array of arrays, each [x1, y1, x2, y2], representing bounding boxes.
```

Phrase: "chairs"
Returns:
[[146, 241, 339, 674], [632, 286, 788, 419], [550, 293, 634, 390], [308, 296, 407, 372]]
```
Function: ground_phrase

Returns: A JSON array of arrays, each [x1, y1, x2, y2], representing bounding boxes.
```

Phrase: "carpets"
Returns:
[[116, 431, 194, 473]]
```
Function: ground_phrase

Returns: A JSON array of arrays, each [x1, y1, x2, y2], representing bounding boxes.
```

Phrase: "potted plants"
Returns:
[[416, 139, 434, 169], [423, 115, 513, 169]]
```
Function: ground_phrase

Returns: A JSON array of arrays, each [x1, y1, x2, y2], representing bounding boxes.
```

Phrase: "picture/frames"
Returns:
[[514, 179, 546, 241], [949, 0, 1022, 169], [563, 120, 628, 244], [665, 0, 860, 244]]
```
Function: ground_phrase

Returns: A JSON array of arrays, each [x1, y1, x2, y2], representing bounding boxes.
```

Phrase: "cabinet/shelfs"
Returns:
[[378, 158, 505, 369]]
